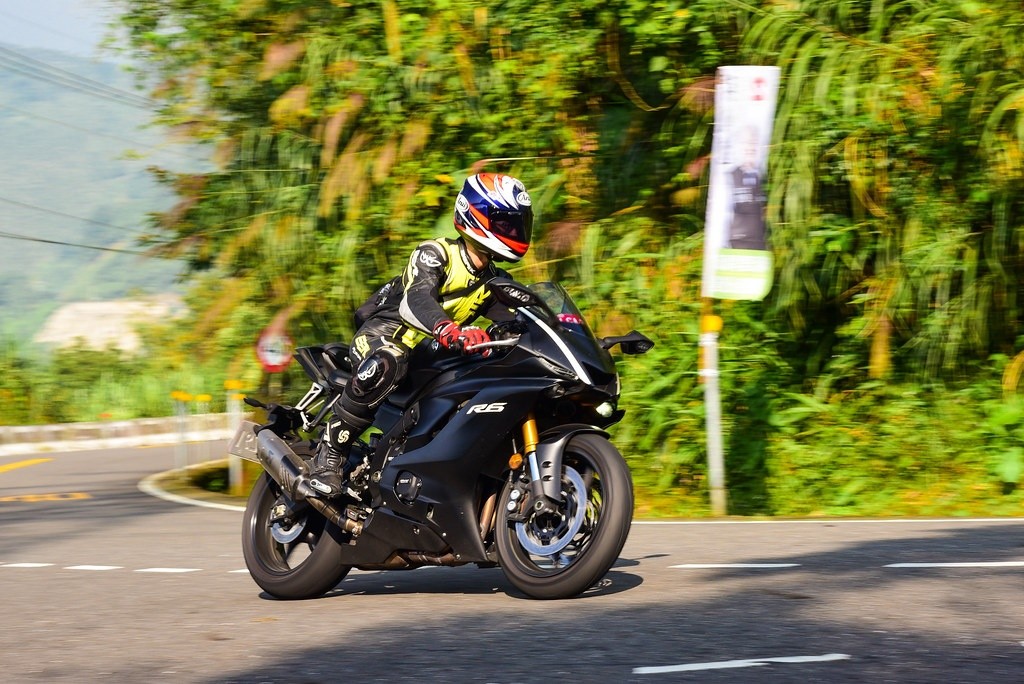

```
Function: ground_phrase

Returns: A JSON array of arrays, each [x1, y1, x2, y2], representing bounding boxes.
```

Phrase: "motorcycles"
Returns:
[[238, 275, 657, 600]]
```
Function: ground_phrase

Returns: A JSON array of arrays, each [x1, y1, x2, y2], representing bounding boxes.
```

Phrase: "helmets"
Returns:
[[454, 173, 534, 263]]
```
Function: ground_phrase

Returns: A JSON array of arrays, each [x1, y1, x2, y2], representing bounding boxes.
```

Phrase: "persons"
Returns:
[[307, 174, 534, 497]]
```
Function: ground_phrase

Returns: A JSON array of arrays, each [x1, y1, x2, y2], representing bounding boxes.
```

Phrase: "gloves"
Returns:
[[433, 319, 492, 358]]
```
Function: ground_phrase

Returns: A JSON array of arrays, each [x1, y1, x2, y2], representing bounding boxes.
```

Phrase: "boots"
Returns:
[[307, 403, 373, 502]]
